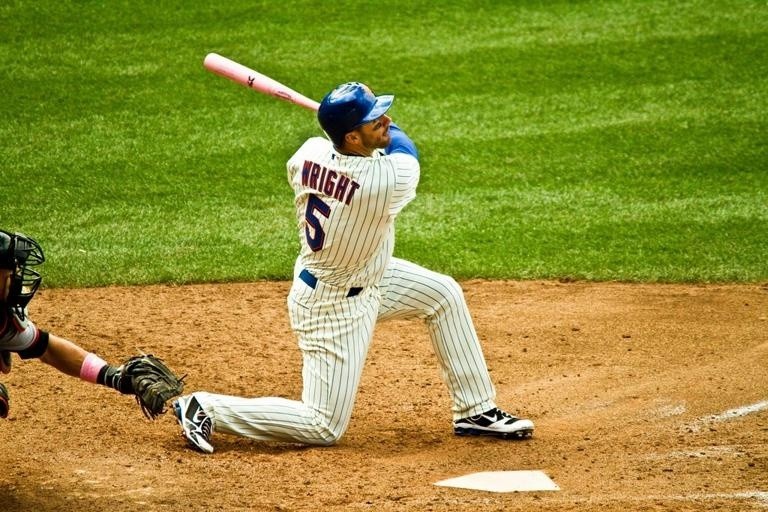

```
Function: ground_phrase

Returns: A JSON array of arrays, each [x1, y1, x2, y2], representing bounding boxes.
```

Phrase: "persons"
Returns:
[[169, 81, 537, 456], [0, 229, 185, 418]]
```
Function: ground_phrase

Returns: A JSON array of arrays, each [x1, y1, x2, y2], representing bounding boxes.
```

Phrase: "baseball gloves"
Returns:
[[111, 348, 188, 419]]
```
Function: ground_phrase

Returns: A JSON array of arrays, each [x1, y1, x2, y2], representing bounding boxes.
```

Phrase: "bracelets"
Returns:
[[80, 352, 108, 383]]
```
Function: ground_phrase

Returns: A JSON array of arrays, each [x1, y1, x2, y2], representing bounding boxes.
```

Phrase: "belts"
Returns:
[[299, 269, 363, 296]]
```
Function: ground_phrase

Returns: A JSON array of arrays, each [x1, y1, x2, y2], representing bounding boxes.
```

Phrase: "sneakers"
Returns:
[[452, 406, 534, 438], [171, 391, 214, 454]]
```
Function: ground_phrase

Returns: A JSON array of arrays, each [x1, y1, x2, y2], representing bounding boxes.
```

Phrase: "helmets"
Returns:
[[0, 229, 45, 320], [317, 81, 395, 146]]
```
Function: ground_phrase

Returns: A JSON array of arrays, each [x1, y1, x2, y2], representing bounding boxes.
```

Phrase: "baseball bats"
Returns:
[[203, 52, 321, 111]]
